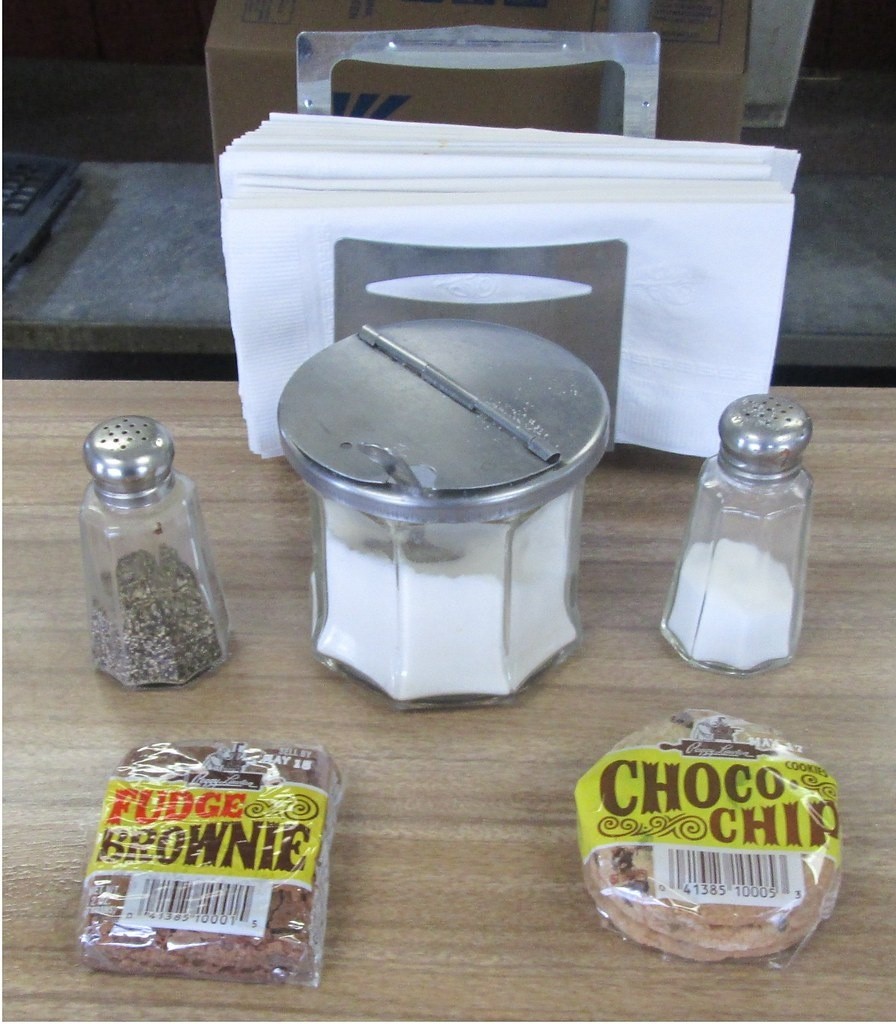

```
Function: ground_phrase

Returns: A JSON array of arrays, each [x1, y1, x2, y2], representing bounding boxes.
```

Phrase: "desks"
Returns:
[[2, 378, 896, 1023]]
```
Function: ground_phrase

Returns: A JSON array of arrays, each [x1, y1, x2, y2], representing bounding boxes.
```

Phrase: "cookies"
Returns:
[[575, 708, 845, 960], [74, 738, 343, 986]]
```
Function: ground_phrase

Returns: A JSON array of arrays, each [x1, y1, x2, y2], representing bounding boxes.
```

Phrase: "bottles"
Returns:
[[660, 395, 811, 674], [78, 415, 232, 691]]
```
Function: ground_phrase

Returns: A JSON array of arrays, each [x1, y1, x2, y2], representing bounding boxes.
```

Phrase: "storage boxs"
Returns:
[[205, 0, 756, 206]]
